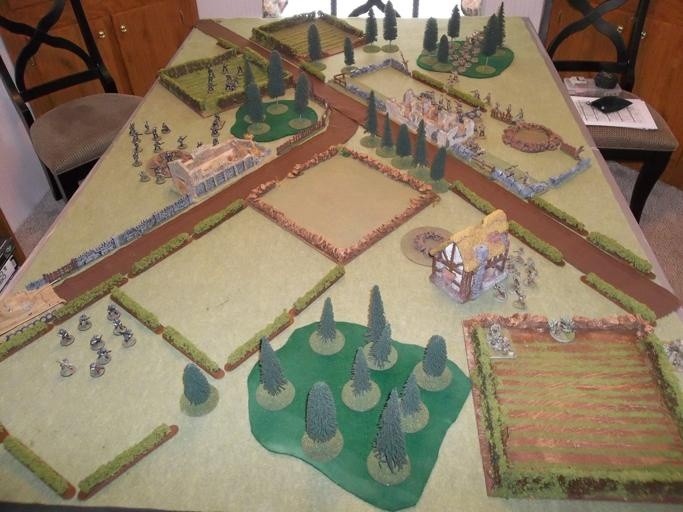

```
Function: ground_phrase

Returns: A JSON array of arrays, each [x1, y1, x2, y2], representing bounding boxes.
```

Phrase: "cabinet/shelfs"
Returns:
[[0, 0, 200, 118]]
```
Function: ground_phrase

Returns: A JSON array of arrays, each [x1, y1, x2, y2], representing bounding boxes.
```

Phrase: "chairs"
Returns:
[[0, 0, 144, 201], [539, 0, 678, 224]]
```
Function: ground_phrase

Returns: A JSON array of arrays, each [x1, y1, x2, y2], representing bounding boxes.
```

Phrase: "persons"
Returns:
[[429, 82, 527, 139], [494, 245, 541, 307], [205, 59, 244, 93], [54, 303, 133, 375], [128, 114, 222, 183]]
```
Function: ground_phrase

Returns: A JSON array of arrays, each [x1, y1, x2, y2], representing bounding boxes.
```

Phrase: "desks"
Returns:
[[0, 18, 683, 512]]
